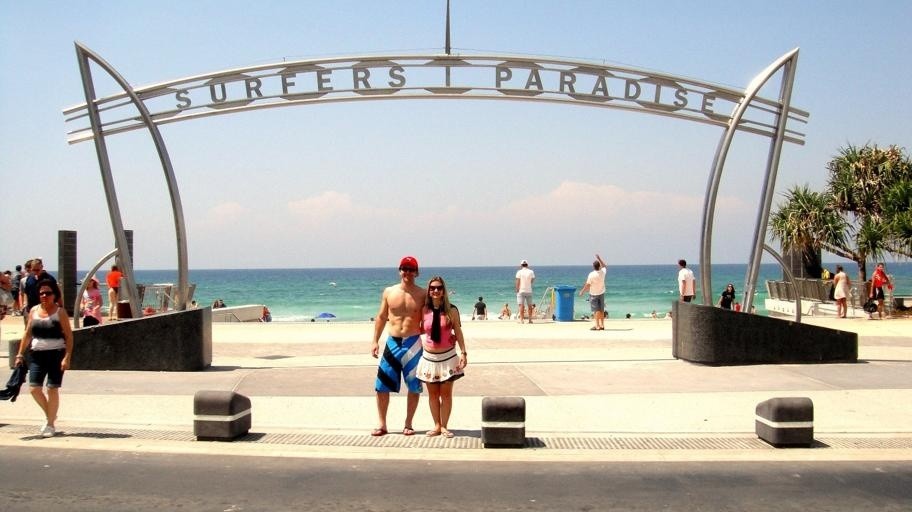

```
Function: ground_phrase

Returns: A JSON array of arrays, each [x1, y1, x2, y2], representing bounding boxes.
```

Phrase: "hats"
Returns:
[[79, 273, 99, 285], [521, 260, 528, 265], [399, 256, 418, 269]]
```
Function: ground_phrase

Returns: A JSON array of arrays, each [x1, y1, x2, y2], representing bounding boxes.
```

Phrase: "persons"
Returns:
[[867, 263, 892, 320], [0, 259, 127, 330], [372, 257, 458, 435], [578, 254, 742, 330], [416, 276, 467, 437], [831, 261, 852, 319], [472, 260, 536, 323], [191, 299, 272, 323], [15, 280, 73, 438]]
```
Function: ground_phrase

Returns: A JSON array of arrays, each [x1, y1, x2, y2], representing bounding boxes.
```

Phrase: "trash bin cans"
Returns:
[[551, 286, 578, 321]]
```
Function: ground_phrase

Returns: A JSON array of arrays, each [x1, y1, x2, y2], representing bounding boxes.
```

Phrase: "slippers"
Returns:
[[371, 428, 388, 436], [590, 327, 604, 330], [403, 428, 415, 435], [426, 430, 455, 438]]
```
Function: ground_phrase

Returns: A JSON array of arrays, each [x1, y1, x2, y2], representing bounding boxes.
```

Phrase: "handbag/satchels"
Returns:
[[829, 283, 837, 301], [863, 298, 878, 312]]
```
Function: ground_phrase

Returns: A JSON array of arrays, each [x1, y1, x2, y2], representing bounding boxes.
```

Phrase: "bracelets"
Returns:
[[16, 354, 24, 358], [460, 352, 467, 356]]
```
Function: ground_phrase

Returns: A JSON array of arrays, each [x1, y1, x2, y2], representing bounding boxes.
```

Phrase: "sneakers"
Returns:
[[41, 415, 57, 437]]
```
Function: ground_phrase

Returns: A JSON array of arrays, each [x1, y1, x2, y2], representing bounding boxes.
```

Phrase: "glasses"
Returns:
[[39, 291, 54, 295], [429, 285, 444, 290], [400, 268, 415, 272]]
